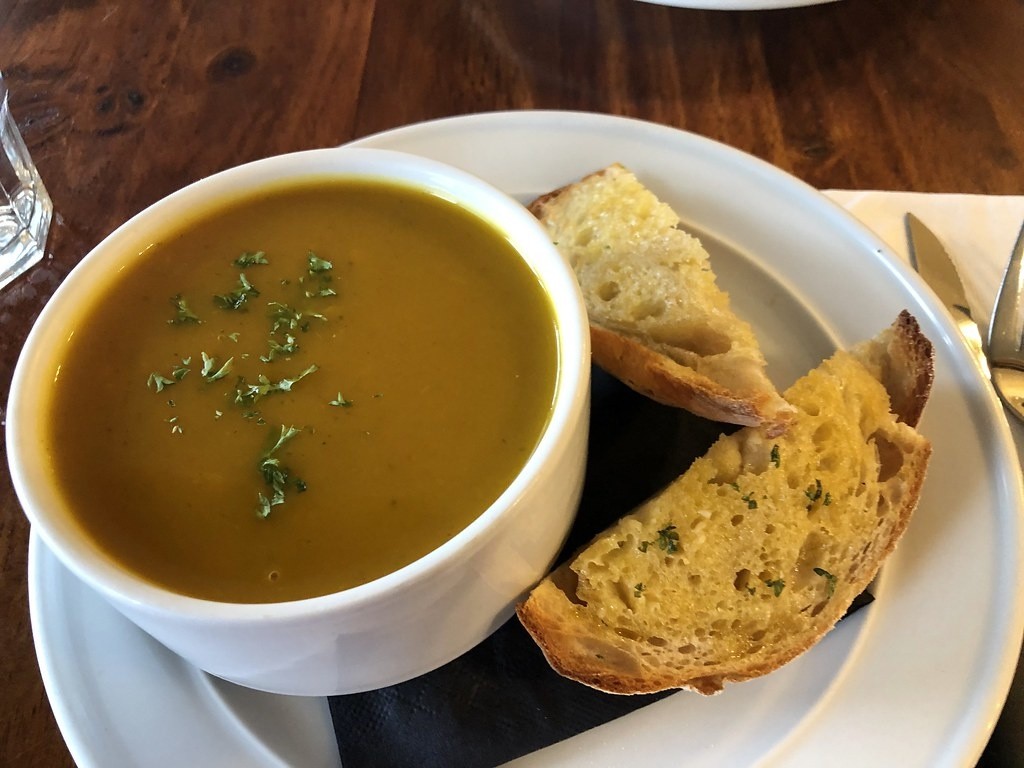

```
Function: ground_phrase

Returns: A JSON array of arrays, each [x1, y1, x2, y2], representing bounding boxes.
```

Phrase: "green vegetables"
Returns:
[[148, 248, 353, 518]]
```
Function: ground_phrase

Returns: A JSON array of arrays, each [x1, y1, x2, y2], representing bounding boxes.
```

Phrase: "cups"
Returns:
[[986, 219, 1024, 425], [0, 68, 54, 291]]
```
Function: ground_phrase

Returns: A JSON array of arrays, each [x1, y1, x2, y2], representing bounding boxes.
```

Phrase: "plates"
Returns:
[[27, 109, 1024, 768]]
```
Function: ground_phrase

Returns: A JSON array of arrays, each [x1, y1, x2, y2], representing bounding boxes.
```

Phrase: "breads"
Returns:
[[514, 310, 936, 698], [526, 161, 797, 438]]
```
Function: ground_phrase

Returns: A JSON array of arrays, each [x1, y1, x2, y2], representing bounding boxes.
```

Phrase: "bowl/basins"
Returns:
[[5, 150, 591, 698]]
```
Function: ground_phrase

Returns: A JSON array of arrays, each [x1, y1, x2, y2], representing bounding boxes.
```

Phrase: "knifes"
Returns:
[[903, 212, 991, 382]]
